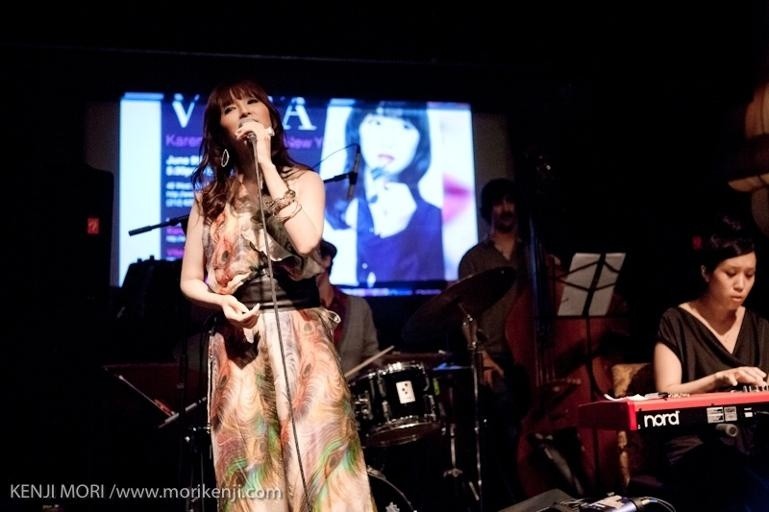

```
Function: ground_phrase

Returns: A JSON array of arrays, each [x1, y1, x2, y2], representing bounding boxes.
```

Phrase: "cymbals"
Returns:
[[404, 266, 515, 344], [431, 363, 470, 373]]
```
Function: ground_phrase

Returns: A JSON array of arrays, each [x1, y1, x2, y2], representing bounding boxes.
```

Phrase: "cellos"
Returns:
[[496, 149, 661, 499]]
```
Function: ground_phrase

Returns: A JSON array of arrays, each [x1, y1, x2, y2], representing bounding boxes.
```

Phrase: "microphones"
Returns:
[[241, 118, 256, 143], [347, 146, 361, 200]]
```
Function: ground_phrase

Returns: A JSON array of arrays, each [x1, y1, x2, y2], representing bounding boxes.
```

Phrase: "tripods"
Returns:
[[414, 374, 482, 512]]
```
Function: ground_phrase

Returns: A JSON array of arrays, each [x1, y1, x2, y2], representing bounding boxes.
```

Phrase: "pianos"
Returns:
[[577, 383, 767, 434]]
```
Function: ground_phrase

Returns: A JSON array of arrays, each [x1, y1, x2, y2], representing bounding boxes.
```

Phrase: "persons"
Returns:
[[451, 175, 550, 392], [651, 218, 768, 511], [321, 100, 445, 287], [176, 80, 377, 512], [316, 238, 381, 382]]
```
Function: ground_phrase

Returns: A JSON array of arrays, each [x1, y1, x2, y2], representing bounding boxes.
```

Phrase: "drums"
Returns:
[[364, 466, 419, 512], [346, 361, 448, 449]]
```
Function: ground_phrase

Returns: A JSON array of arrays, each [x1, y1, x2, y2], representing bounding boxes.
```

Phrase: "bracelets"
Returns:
[[266, 189, 296, 215], [275, 203, 302, 223]]
